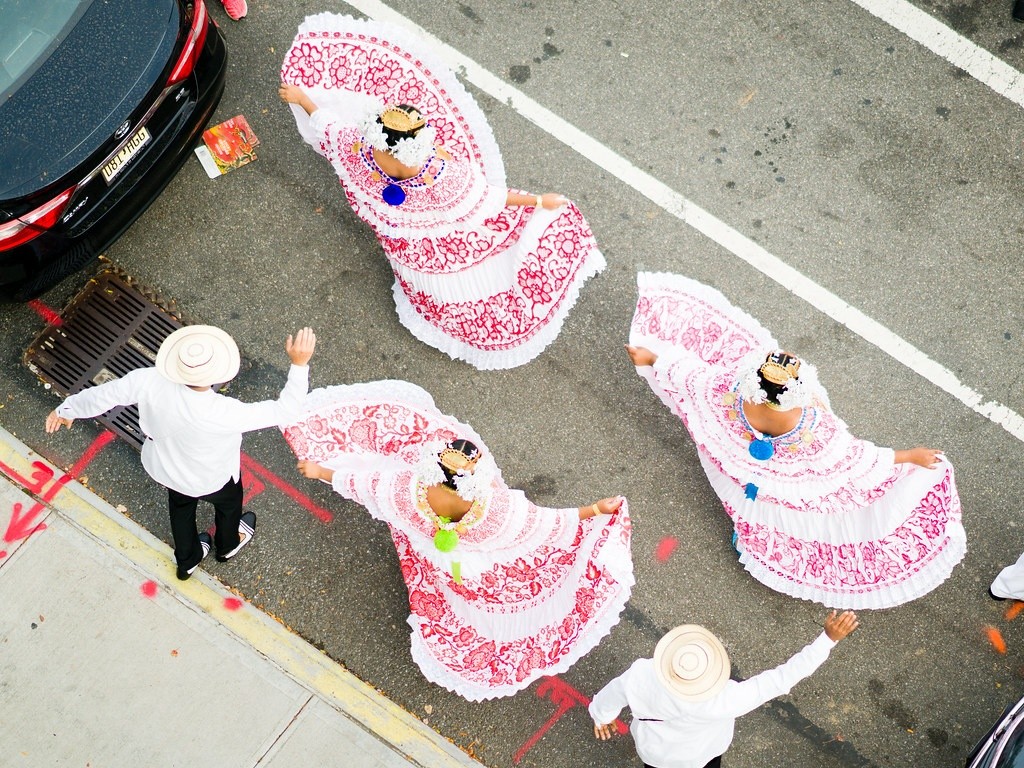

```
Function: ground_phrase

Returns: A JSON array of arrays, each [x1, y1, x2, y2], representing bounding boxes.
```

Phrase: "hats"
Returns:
[[155, 323, 242, 387], [652, 625, 731, 704]]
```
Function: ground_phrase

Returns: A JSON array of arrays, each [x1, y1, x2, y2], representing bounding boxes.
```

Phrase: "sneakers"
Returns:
[[220, 0, 248, 21]]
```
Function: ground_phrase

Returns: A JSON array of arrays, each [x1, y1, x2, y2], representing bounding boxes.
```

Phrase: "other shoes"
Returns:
[[216, 511, 256, 563], [178, 532, 212, 579]]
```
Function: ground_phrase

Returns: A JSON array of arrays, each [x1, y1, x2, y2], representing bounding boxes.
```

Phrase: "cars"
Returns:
[[0, 0, 231, 309]]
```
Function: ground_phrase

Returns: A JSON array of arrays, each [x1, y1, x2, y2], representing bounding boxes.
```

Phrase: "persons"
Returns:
[[278, 12, 606, 369], [988, 553, 1024, 602], [588, 608, 858, 768], [47, 326, 316, 580], [280, 380, 636, 704], [624, 272, 967, 609], [222, 0, 248, 20]]
